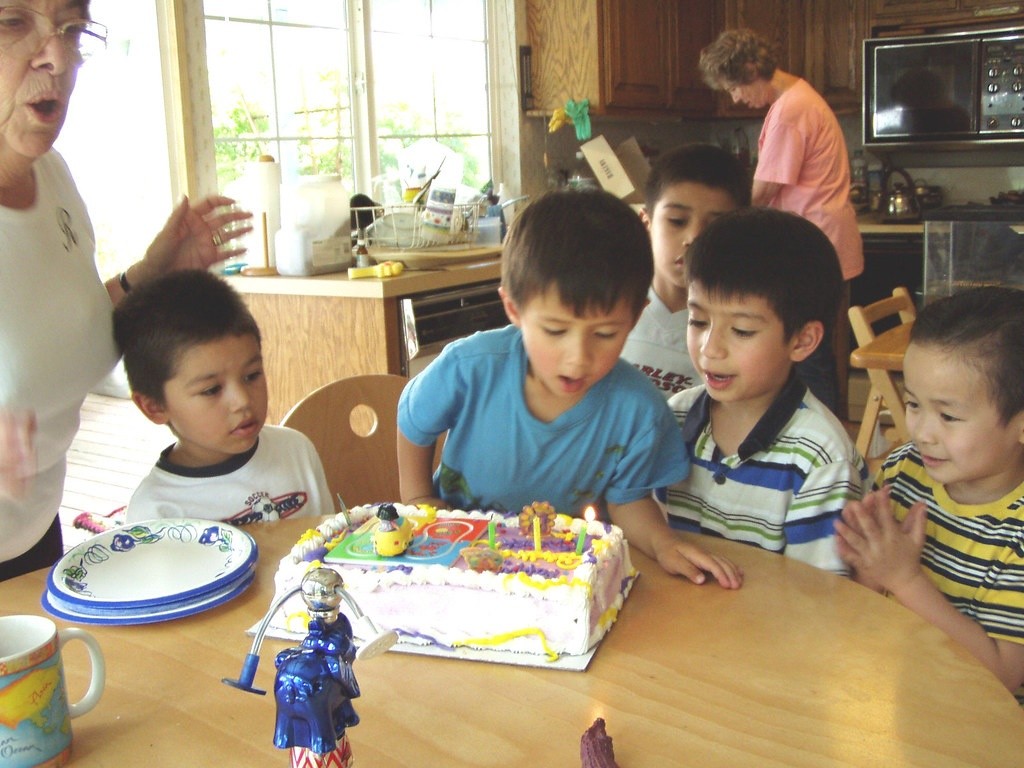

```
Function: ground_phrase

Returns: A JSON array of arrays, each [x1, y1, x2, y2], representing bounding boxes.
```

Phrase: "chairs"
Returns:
[[280, 375, 449, 507], [846, 286, 927, 469]]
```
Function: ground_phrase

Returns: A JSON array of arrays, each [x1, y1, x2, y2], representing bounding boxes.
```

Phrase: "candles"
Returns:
[[575, 509, 597, 556], [530, 512, 546, 562], [485, 510, 498, 555]]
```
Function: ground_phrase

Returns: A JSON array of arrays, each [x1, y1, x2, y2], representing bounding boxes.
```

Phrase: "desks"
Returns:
[[1, 513, 1024, 768], [849, 323, 915, 371]]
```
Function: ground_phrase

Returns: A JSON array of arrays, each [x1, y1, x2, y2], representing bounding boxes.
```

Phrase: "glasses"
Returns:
[[0, 6, 109, 69]]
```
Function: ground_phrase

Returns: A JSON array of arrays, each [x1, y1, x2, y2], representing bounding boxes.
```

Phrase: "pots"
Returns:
[[903, 178, 943, 207]]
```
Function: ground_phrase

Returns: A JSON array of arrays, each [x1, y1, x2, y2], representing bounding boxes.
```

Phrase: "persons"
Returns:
[[653, 207, 873, 578], [618, 144, 751, 402], [397, 189, 744, 590], [112, 270, 337, 527], [832, 285, 1024, 710], [697, 29, 863, 421], [0, 0, 254, 581]]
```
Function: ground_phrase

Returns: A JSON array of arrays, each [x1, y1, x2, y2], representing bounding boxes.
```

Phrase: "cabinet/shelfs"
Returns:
[[523, 0, 870, 119]]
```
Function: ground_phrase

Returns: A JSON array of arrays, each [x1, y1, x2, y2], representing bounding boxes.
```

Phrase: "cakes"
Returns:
[[271, 501, 638, 663]]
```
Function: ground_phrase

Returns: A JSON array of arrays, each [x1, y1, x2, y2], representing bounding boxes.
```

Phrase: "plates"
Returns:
[[41, 517, 258, 625]]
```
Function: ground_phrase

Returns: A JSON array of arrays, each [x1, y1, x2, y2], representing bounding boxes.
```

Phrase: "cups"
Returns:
[[421, 188, 456, 242], [0, 614, 105, 768]]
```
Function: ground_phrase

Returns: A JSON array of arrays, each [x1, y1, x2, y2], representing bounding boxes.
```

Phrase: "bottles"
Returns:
[[567, 151, 598, 193], [356, 239, 369, 267], [850, 150, 869, 201], [498, 184, 514, 227], [405, 187, 426, 208]]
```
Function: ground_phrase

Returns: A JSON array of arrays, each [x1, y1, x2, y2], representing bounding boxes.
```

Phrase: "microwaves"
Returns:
[[862, 28, 1024, 147]]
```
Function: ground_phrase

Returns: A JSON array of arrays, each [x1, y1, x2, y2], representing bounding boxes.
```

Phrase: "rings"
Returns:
[[212, 231, 223, 247]]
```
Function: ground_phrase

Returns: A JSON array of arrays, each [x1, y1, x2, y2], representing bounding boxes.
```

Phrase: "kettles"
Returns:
[[878, 167, 920, 224]]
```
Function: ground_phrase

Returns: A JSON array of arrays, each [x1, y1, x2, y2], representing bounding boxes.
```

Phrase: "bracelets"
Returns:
[[119, 273, 131, 294]]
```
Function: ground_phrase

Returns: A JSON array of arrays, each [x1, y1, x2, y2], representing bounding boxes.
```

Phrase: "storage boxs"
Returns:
[[923, 203, 1023, 308]]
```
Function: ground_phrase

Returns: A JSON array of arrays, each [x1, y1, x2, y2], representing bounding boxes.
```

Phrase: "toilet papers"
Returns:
[[243, 161, 283, 267]]
